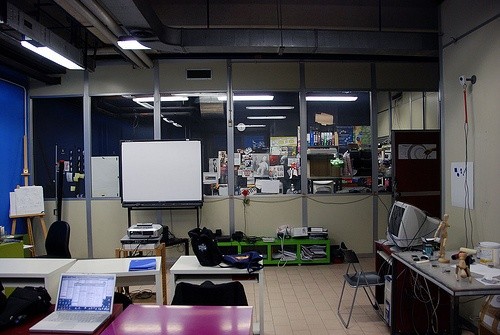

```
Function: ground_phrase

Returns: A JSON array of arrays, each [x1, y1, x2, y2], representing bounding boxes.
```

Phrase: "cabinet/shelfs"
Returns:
[[218, 239, 330, 264], [374, 241, 452, 335]]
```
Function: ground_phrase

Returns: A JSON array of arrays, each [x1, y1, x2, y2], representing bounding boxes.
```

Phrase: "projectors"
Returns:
[[126, 224, 163, 238]]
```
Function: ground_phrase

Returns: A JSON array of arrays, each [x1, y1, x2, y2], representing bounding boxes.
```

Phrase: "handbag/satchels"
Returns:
[[188, 227, 223, 266], [217, 251, 264, 274]]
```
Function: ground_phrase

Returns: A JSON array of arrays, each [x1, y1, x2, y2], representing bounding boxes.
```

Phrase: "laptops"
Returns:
[[28, 273, 116, 334]]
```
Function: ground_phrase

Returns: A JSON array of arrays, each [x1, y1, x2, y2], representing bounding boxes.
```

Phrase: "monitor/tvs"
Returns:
[[386, 201, 441, 247]]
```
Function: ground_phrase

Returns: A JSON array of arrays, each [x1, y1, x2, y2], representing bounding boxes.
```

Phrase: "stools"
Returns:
[[9, 213, 47, 256], [114, 242, 167, 305]]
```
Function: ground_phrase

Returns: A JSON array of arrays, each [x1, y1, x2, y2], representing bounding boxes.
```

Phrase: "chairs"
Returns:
[[337, 242, 383, 328], [24, 221, 72, 259]]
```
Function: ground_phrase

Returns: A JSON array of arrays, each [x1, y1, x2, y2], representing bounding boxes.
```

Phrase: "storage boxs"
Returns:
[[384, 275, 393, 326]]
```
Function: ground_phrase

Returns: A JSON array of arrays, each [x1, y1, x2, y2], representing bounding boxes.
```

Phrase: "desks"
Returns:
[[0, 242, 264, 335], [392, 251, 500, 335]]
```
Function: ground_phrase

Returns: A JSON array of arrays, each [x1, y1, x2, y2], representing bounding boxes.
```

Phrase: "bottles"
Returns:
[[290, 184, 294, 192], [479, 241, 500, 265]]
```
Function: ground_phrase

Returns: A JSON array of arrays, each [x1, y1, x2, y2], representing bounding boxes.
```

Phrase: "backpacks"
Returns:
[[0, 286, 53, 327]]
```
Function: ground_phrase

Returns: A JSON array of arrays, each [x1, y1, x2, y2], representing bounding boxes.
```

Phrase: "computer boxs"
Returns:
[[383, 275, 393, 326]]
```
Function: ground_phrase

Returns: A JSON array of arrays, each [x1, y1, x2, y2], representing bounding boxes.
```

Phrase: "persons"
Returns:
[[451, 250, 475, 282], [434, 214, 452, 258]]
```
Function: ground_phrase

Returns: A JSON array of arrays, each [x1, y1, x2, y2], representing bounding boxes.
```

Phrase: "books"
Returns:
[[301, 245, 326, 260]]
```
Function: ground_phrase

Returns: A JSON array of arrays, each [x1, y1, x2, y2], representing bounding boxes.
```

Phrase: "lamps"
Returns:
[[117, 33, 152, 50]]
